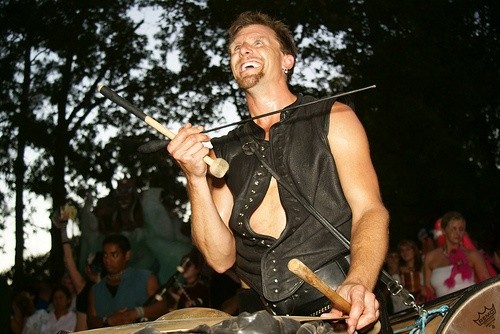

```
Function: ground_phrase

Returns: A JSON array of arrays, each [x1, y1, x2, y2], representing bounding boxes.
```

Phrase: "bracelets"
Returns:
[[63, 240, 70, 244]]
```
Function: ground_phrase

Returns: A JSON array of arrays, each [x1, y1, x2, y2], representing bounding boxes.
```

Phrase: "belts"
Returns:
[[258, 256, 352, 316]]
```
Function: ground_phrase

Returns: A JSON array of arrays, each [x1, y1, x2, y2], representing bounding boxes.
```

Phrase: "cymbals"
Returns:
[[67, 308, 350, 334]]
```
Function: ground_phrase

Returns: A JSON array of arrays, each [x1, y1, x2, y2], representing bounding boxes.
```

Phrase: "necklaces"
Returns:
[[443, 241, 462, 257]]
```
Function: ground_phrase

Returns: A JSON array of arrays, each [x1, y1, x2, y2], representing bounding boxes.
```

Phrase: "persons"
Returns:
[[0, 211, 262, 334], [166, 11, 390, 334], [381, 211, 500, 312]]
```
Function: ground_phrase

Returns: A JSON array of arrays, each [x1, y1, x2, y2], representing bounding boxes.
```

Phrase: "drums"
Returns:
[[418, 275, 500, 334]]
